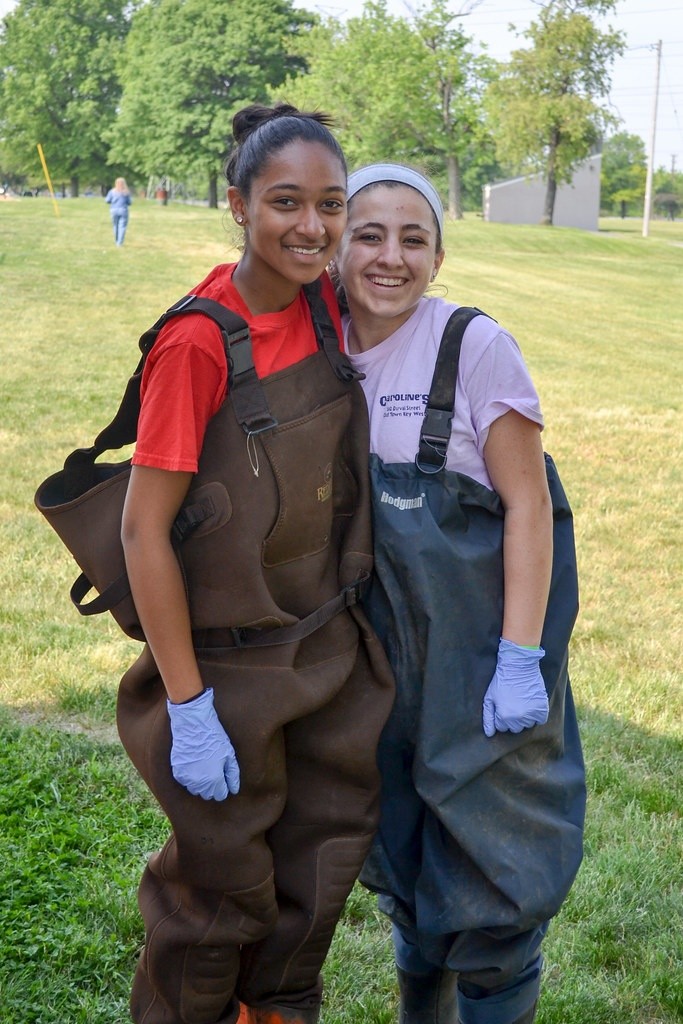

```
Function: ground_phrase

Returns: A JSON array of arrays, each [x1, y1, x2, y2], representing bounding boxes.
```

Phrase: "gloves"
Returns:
[[164, 688, 242, 804], [479, 637, 550, 738]]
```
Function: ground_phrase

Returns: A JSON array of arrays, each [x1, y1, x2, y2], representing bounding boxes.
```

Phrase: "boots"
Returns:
[[396, 962, 459, 1024]]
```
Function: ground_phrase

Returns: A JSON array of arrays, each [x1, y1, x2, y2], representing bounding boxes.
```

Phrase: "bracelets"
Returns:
[[179, 687, 206, 705], [520, 645, 539, 650]]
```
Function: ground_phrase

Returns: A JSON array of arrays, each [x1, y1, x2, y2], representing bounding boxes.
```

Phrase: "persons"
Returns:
[[117, 104, 396, 1024], [105, 178, 132, 246], [328, 161, 588, 1024]]
